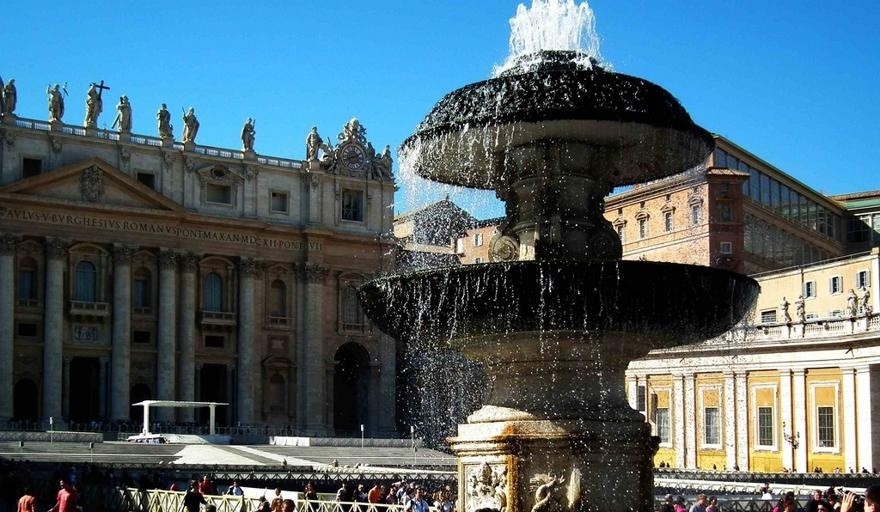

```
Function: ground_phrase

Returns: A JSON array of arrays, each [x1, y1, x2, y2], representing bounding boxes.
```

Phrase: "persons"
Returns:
[[115, 95, 132, 134], [860, 285, 871, 312], [2, 79, 18, 115], [85, 85, 104, 124], [183, 108, 199, 143], [240, 117, 257, 152], [155, 103, 173, 138], [46, 83, 64, 122], [306, 126, 324, 161], [794, 294, 806, 323], [781, 297, 792, 323], [85, 417, 296, 437], [15, 461, 880, 511], [847, 289, 858, 316]]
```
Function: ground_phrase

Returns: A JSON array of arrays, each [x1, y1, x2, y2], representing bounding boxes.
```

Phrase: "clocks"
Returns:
[[341, 143, 365, 171]]
